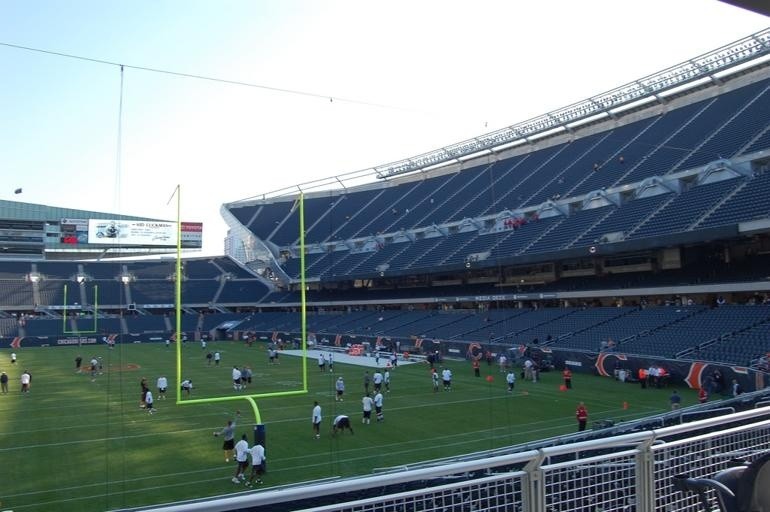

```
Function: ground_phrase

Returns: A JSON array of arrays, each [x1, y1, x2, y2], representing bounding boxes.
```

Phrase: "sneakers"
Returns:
[[238, 474, 245, 480], [245, 482, 253, 488], [233, 455, 236, 461], [316, 433, 320, 439], [232, 478, 240, 484], [255, 480, 263, 484]]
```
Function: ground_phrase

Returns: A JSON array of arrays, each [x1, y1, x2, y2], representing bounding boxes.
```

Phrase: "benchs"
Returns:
[[227, 81, 770, 368], [3, 253, 277, 342]]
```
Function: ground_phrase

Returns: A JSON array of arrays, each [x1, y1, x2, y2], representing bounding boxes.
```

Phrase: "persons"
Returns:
[[1, 371, 9, 395], [112, 338, 116, 348], [91, 356, 97, 375], [335, 376, 345, 402], [96, 356, 105, 375], [108, 339, 111, 349], [505, 215, 540, 231], [345, 341, 401, 425], [182, 335, 188, 347], [755, 353, 765, 369], [244, 439, 267, 489], [20, 370, 30, 394], [26, 369, 33, 392], [72, 353, 83, 374], [613, 357, 672, 390], [213, 412, 241, 463], [10, 350, 18, 367], [697, 366, 722, 402], [426, 342, 532, 394], [328, 353, 334, 373], [669, 390, 683, 410], [731, 378, 743, 397], [230, 432, 250, 485], [105, 221, 122, 238], [199, 332, 329, 367], [318, 353, 326, 372], [231, 363, 253, 392], [164, 337, 170, 349], [89, 362, 97, 382], [576, 400, 589, 432], [563, 366, 572, 389], [762, 353, 770, 373], [312, 401, 322, 439], [331, 414, 355, 435], [138, 372, 196, 416]]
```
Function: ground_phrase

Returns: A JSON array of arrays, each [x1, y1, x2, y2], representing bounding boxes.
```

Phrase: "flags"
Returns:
[[14, 188, 22, 195]]
